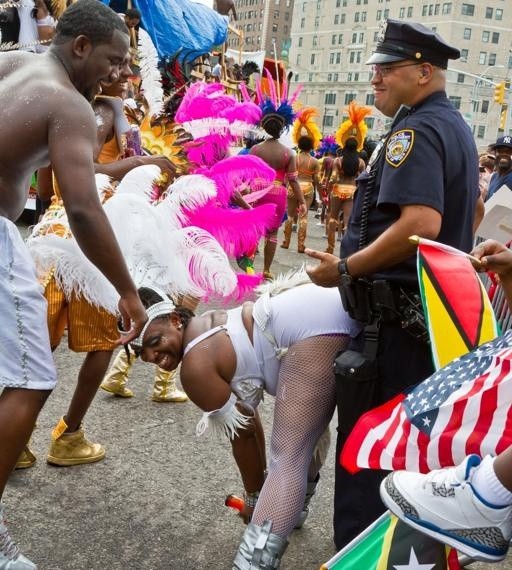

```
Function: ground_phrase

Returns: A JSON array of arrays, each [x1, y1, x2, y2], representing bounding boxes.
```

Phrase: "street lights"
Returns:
[[456, 55, 480, 136], [474, 63, 506, 131]]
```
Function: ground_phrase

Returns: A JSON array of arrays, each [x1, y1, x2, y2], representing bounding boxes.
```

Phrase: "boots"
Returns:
[[150, 363, 190, 406], [279, 219, 293, 250], [323, 218, 339, 254], [288, 473, 321, 532], [229, 519, 290, 570], [99, 347, 138, 399], [297, 217, 307, 254]]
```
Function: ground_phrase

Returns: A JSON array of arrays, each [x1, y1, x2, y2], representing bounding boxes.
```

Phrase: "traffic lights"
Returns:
[[499, 109, 504, 128], [494, 79, 502, 104]]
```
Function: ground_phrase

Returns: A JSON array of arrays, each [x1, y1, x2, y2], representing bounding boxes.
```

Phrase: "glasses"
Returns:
[[370, 61, 423, 74]]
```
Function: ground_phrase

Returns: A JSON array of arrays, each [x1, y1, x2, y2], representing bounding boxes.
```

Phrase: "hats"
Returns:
[[493, 135, 512, 151], [364, 16, 460, 71]]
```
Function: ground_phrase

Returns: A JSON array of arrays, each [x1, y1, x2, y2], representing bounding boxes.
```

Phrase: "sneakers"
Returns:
[[373, 452, 512, 568], [45, 425, 105, 467], [13, 420, 39, 469], [263, 270, 274, 280], [0, 519, 38, 570]]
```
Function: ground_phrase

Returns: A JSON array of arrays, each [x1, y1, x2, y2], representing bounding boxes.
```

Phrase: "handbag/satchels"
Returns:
[[332, 348, 390, 435]]
